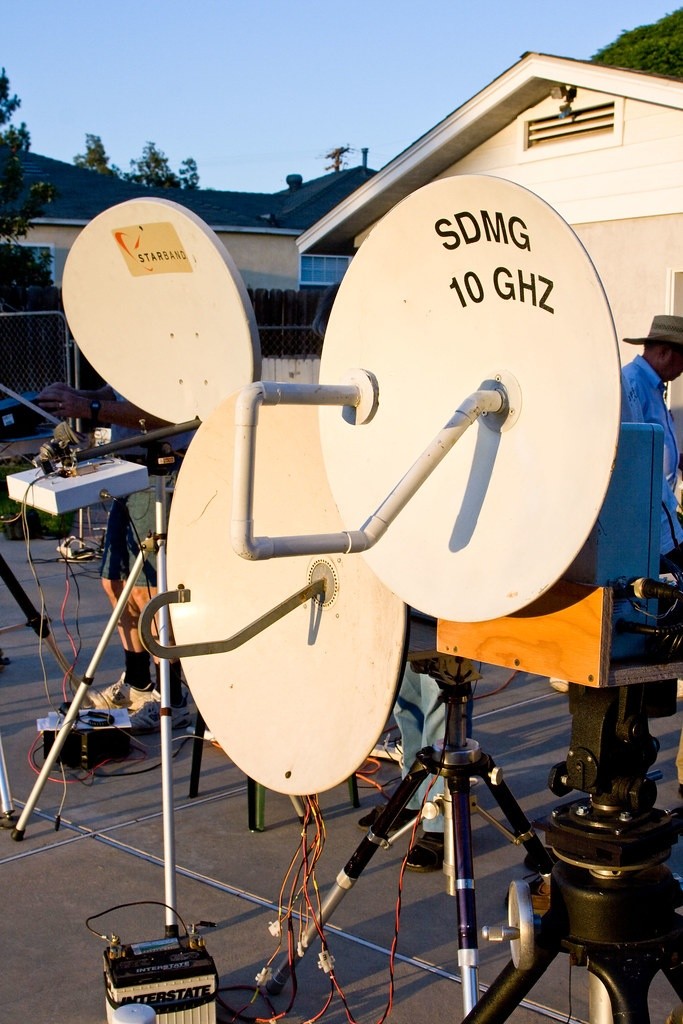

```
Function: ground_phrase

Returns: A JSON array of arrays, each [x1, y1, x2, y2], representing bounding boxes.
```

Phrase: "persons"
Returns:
[[622, 316, 683, 573], [36, 376, 194, 730], [357, 609, 473, 874]]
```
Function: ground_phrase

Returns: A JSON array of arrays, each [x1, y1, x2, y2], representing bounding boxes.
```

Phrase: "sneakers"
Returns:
[[130, 693, 191, 736], [83, 670, 156, 711]]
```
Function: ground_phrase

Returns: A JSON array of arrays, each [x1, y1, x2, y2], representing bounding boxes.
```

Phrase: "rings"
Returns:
[[59, 403, 62, 407]]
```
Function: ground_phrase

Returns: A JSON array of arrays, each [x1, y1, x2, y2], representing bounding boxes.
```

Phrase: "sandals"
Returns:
[[402, 838, 446, 872], [356, 803, 417, 836], [550, 678, 570, 692]]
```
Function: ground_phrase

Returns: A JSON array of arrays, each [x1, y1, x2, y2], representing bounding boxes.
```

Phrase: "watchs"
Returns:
[[89, 399, 102, 420]]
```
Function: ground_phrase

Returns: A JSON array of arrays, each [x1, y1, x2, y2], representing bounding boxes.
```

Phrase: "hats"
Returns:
[[623, 314, 683, 343]]
